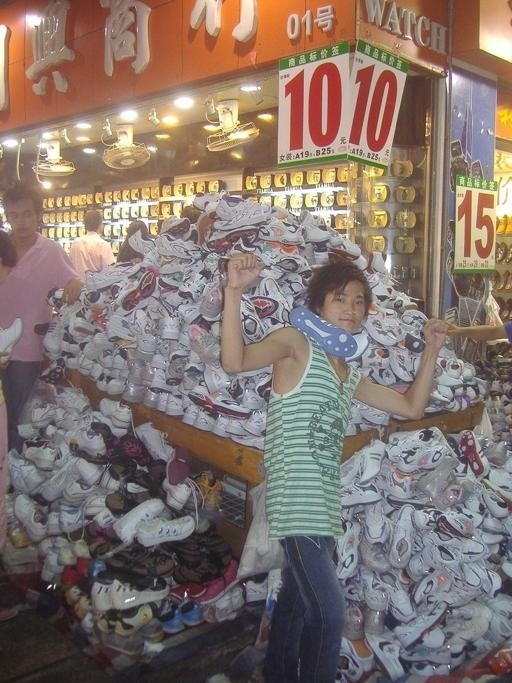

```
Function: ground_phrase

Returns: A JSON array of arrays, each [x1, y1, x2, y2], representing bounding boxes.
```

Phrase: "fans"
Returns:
[[103, 124, 150, 170], [206, 99, 260, 152], [32, 138, 75, 177]]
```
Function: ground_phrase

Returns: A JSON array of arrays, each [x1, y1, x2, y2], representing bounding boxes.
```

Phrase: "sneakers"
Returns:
[[1, 317, 22, 370], [33, 189, 487, 453], [0, 424, 288, 656], [487, 215, 512, 454], [333, 453, 512, 683]]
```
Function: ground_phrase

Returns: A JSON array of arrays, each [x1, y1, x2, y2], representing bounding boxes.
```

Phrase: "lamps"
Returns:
[[148, 105, 160, 127]]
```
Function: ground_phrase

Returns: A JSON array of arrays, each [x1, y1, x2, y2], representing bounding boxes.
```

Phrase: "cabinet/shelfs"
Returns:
[[35, 356, 485, 570]]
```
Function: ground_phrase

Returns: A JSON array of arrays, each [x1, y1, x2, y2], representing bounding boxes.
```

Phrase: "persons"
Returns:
[[117, 220, 155, 263], [220, 252, 451, 683], [0, 184, 82, 561], [445, 318, 512, 344], [70, 209, 116, 277]]
